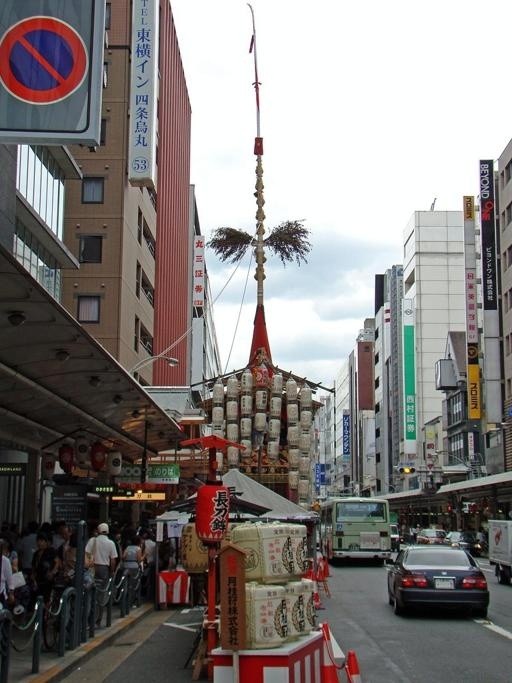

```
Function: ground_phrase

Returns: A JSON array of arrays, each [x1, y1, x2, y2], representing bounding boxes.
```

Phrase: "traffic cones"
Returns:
[[322, 622, 340, 682], [310, 569, 325, 610], [305, 556, 330, 579], [347, 650, 362, 682]]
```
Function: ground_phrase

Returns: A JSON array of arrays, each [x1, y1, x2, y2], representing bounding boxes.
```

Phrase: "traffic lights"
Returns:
[[393, 465, 417, 476], [448, 505, 452, 512]]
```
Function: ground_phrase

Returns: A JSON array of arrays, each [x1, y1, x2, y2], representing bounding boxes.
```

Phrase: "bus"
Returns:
[[316, 497, 391, 565]]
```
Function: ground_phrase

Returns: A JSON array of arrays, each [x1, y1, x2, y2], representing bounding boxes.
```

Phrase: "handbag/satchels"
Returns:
[[88, 563, 95, 577], [12, 568, 26, 587]]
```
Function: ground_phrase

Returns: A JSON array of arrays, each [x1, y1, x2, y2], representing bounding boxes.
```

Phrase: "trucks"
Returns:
[[488, 519, 512, 584], [389, 510, 405, 543]]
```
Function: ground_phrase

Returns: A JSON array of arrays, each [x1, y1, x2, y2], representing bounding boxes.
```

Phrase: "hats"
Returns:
[[98, 523, 110, 534]]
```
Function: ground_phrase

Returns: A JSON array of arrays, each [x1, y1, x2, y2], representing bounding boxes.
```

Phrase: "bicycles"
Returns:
[[41, 569, 100, 650]]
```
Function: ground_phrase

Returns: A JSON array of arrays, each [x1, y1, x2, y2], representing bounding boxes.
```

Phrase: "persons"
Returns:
[[409, 520, 490, 554], [0, 518, 177, 637]]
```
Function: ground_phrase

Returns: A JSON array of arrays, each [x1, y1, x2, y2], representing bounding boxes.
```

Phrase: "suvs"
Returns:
[[390, 524, 399, 552]]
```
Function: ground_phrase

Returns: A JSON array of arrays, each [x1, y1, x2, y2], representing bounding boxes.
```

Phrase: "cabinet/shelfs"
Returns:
[[157, 567, 191, 611], [208, 629, 323, 683]]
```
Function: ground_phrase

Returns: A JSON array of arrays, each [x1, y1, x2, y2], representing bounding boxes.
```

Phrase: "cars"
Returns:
[[442, 528, 482, 557], [413, 525, 449, 544], [384, 539, 489, 617]]
